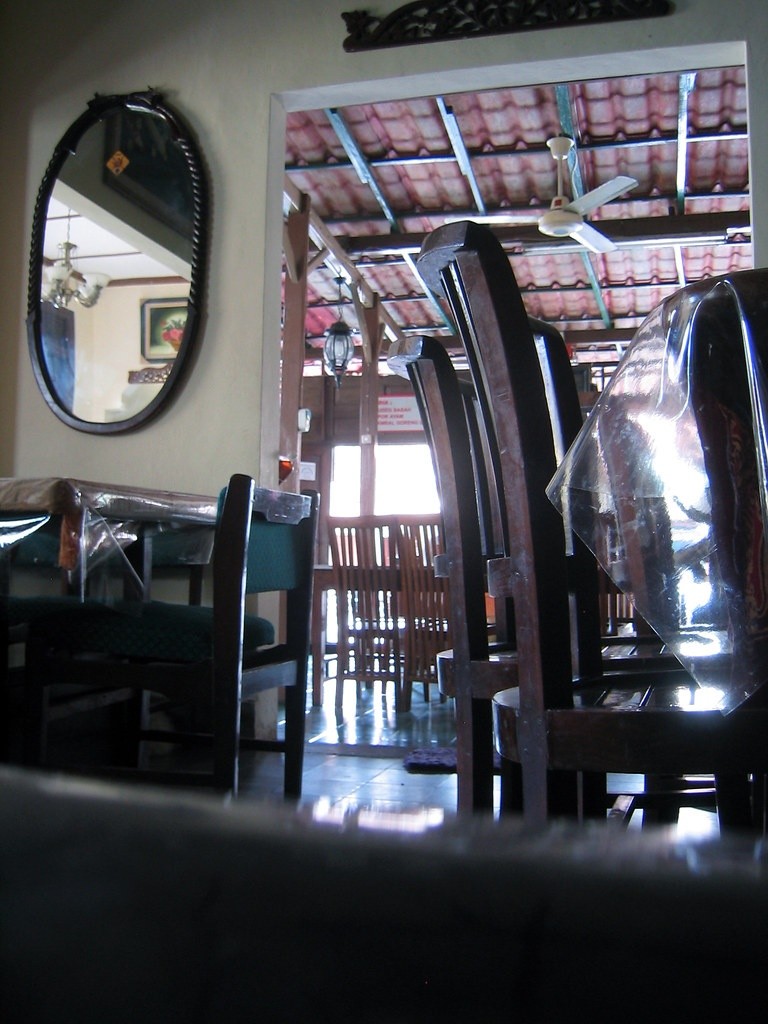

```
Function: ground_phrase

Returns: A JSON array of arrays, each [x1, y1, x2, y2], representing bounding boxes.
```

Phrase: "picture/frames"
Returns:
[[140, 297, 188, 364]]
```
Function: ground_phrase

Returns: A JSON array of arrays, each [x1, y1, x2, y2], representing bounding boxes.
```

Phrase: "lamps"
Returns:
[[40, 207, 110, 309], [323, 276, 356, 389]]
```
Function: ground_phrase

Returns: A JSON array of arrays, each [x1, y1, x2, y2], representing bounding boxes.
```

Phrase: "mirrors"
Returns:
[[22, 83, 213, 436]]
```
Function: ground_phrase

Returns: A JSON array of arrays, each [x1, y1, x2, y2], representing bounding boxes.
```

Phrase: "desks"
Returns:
[[314, 565, 489, 708], [0, 508, 214, 768]]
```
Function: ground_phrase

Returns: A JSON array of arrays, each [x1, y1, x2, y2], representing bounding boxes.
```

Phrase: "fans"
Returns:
[[446, 136, 639, 253]]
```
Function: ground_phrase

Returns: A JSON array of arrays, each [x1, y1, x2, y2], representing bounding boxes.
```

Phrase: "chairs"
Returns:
[[326, 222, 768, 832], [25, 474, 317, 804]]
